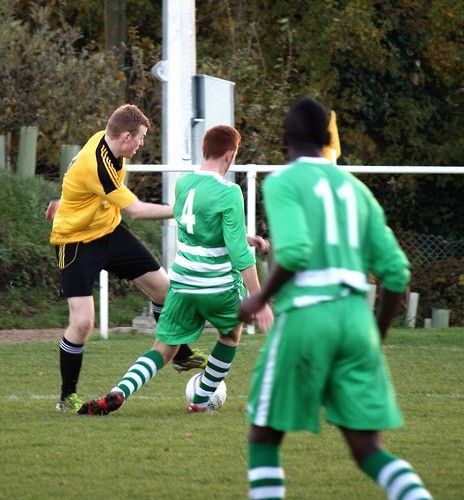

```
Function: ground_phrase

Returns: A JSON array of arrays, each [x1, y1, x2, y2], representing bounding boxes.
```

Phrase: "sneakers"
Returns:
[[188, 402, 219, 416], [174, 352, 209, 372], [56, 392, 85, 413], [79, 390, 125, 416]]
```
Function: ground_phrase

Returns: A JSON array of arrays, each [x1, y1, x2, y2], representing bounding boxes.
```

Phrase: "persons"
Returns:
[[76, 124, 275, 416], [50, 104, 212, 414], [238, 98, 464, 500]]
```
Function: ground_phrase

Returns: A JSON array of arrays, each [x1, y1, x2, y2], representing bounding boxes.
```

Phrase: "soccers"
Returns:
[[185, 372, 228, 411]]
